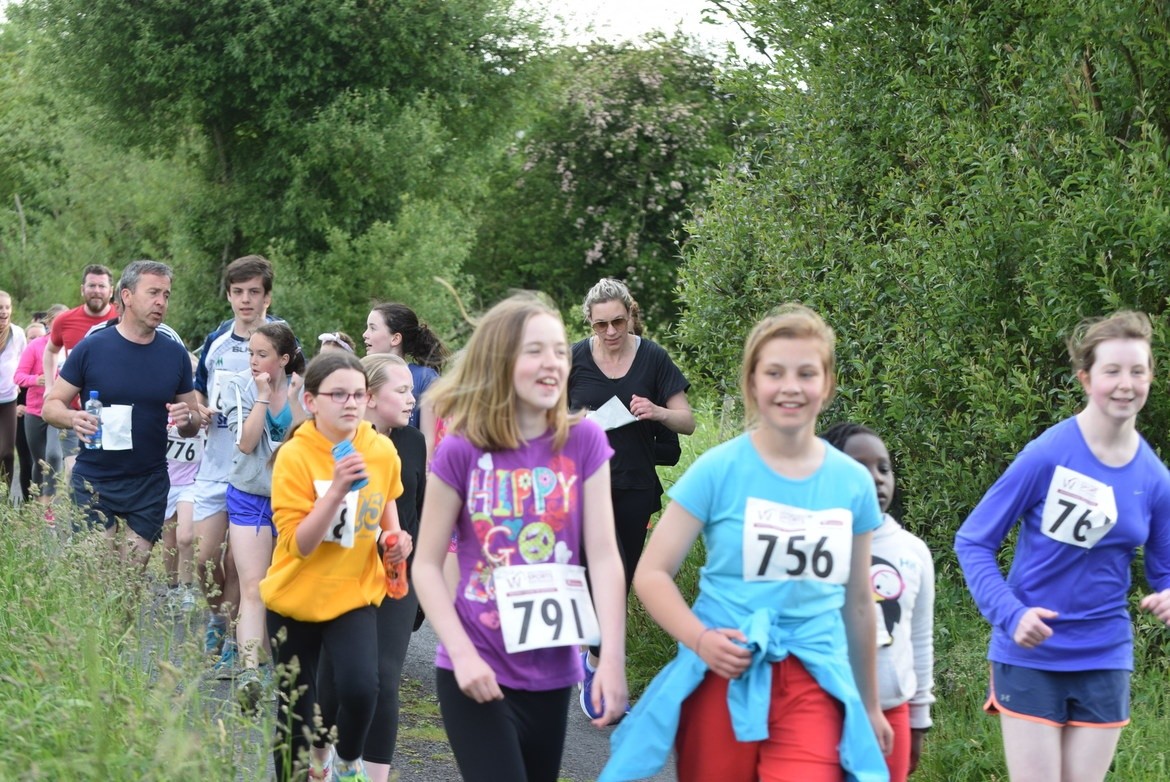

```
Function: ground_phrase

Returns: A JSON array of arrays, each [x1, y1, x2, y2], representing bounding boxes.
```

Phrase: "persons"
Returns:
[[410, 293, 630, 782], [567, 278, 696, 726], [633, 302, 896, 782], [816, 424, 937, 782], [0, 257, 461, 782], [954, 310, 1170, 782]]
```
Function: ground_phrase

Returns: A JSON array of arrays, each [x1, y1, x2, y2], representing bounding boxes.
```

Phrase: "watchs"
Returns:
[[188, 411, 193, 426]]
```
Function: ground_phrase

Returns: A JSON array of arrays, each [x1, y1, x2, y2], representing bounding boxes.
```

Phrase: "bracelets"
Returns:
[[254, 399, 271, 404], [698, 628, 713, 658]]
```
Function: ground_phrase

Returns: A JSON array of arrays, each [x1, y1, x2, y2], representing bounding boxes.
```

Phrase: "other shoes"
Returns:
[[204, 620, 227, 655], [233, 668, 265, 716], [307, 745, 337, 782], [332, 751, 364, 782], [42, 507, 55, 524], [214, 639, 242, 680]]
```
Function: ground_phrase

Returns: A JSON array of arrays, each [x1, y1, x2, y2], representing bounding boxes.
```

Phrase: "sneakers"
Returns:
[[577, 651, 632, 725]]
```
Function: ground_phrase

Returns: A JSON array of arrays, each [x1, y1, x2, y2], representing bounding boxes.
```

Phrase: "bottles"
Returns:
[[384, 535, 410, 600], [85, 390, 103, 449]]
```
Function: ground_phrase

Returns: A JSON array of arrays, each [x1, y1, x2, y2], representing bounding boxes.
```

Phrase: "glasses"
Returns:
[[591, 318, 628, 334], [315, 389, 373, 407]]
